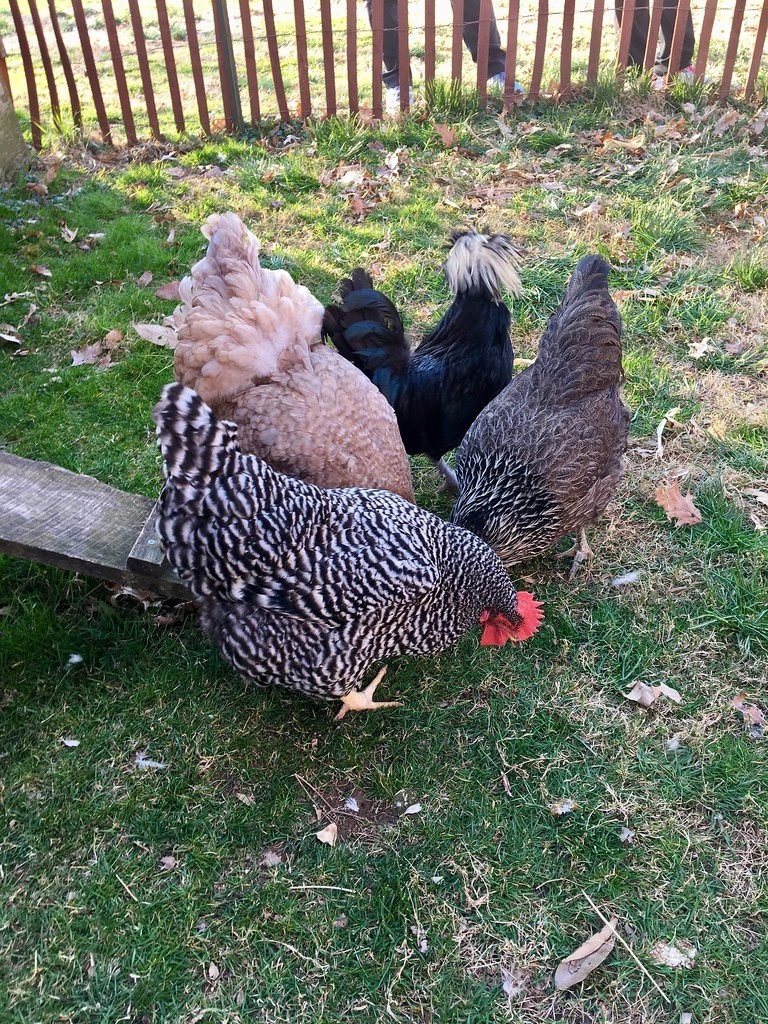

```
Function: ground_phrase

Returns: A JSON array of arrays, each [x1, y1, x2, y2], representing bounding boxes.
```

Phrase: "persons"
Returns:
[[365, 0, 532, 122], [616, 0, 709, 95]]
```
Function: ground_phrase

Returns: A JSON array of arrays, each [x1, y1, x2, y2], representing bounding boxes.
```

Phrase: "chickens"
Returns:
[[170, 210, 413, 508], [149, 378, 545, 723], [324, 224, 531, 498], [449, 251, 636, 585]]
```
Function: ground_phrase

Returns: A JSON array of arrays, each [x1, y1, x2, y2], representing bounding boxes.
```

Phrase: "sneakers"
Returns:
[[487, 72, 526, 93], [651, 64, 700, 88], [386, 86, 414, 118]]
